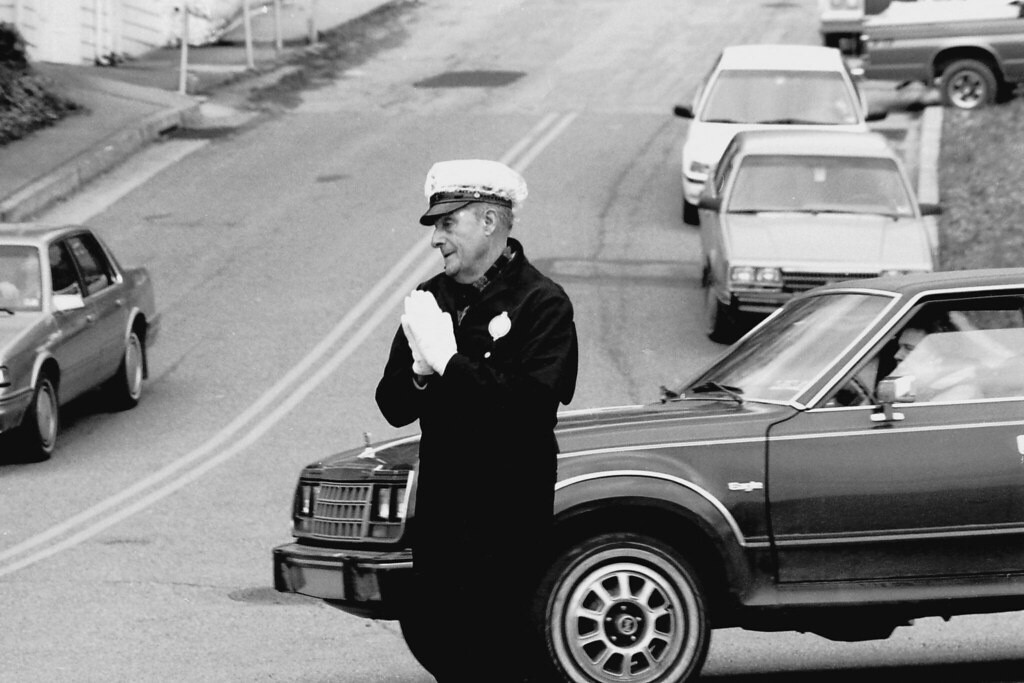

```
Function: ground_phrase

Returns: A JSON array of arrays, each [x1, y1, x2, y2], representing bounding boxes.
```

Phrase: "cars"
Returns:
[[270, 268, 1024, 682], [671, 44, 891, 227], [694, 129, 943, 346], [1, 219, 162, 463]]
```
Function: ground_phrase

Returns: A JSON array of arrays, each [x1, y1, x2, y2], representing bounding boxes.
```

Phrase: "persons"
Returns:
[[375, 159, 579, 683], [847, 321, 984, 404]]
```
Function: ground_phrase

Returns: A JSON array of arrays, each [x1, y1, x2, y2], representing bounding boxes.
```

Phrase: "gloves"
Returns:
[[400, 288, 458, 376], [398, 315, 433, 377]]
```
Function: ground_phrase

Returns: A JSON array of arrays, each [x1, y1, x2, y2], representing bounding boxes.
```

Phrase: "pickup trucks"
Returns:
[[816, 0, 1024, 113]]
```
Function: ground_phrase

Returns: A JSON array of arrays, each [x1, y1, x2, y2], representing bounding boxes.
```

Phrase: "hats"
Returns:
[[418, 158, 528, 226]]
